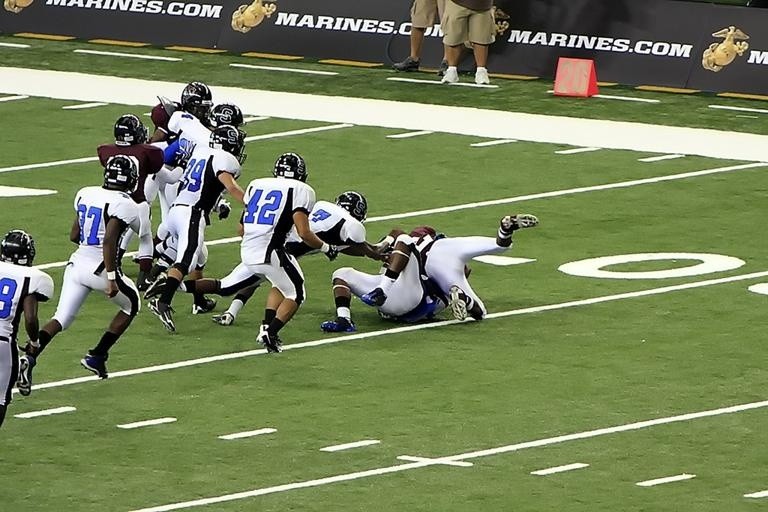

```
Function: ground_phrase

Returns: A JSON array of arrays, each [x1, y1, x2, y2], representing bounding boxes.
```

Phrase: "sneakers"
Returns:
[[192, 297, 216, 315], [393, 56, 420, 71], [449, 285, 468, 321], [501, 214, 539, 232], [360, 288, 388, 306], [255, 324, 284, 353], [81, 350, 107, 379], [211, 310, 233, 326], [320, 317, 357, 332], [437, 60, 459, 83], [16, 356, 36, 396], [136, 264, 176, 331], [474, 67, 489, 84]]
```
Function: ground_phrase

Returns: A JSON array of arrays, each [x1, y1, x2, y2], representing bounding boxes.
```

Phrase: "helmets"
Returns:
[[103, 155, 138, 193], [204, 104, 246, 165], [271, 152, 308, 182], [0, 230, 35, 267], [336, 191, 367, 225], [114, 113, 147, 148], [182, 81, 214, 121]]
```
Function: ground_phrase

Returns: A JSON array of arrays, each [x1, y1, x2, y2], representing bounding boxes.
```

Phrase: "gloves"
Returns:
[[219, 205, 230, 220], [175, 145, 191, 171], [325, 244, 338, 262]]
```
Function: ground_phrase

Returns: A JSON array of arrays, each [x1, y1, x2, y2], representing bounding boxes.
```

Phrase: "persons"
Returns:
[[440, 0, 497, 86], [1, 229, 57, 426], [18, 83, 250, 397], [389, 0, 452, 76], [143, 152, 539, 352]]
